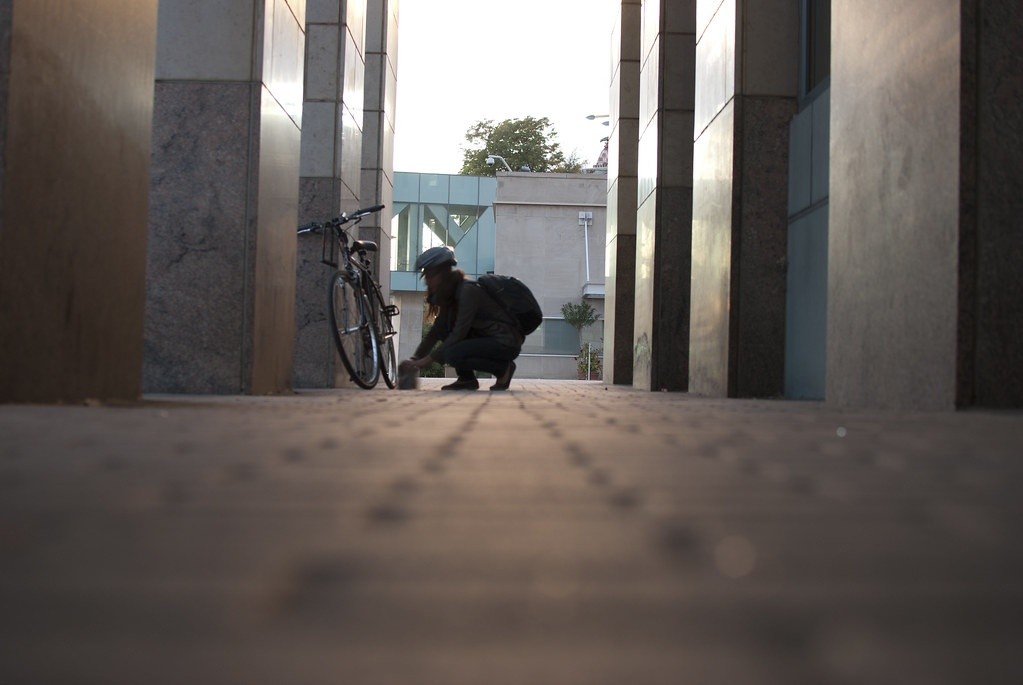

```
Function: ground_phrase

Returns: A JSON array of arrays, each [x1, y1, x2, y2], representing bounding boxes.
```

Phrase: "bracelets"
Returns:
[[410, 357, 417, 360]]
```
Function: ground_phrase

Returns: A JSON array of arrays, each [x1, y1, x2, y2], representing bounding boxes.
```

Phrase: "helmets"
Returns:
[[415, 246, 457, 270]]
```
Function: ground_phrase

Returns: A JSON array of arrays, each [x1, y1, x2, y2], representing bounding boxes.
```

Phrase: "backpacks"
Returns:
[[459, 273, 542, 336]]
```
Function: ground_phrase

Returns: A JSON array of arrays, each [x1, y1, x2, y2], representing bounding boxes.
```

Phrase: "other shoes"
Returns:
[[441, 381, 478, 390], [490, 362, 516, 390]]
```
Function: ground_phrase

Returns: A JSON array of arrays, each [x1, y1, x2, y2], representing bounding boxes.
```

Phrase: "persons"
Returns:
[[401, 246, 526, 391]]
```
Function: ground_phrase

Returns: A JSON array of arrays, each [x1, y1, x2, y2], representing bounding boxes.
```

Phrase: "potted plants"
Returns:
[[574, 343, 603, 381]]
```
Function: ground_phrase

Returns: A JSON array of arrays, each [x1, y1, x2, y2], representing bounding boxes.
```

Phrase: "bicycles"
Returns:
[[293, 203, 401, 391]]
